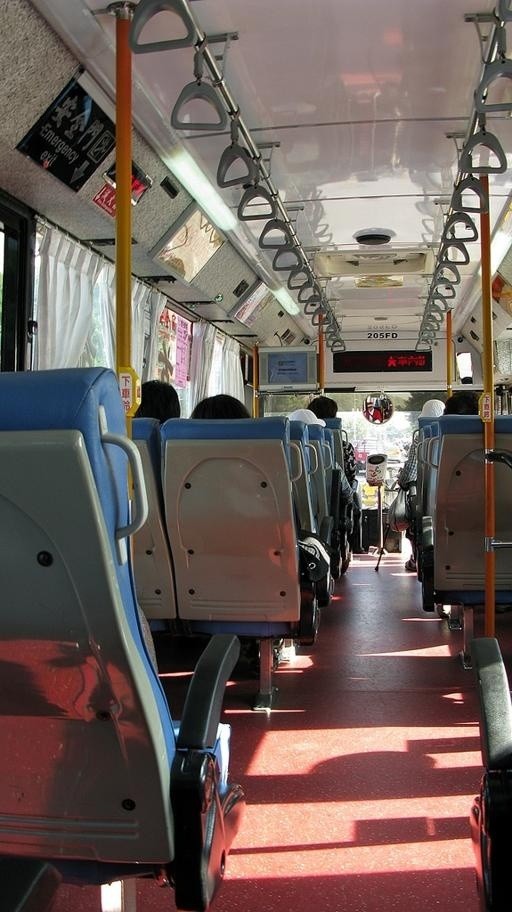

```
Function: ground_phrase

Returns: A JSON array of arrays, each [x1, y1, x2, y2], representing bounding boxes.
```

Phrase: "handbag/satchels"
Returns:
[[388, 488, 412, 532]]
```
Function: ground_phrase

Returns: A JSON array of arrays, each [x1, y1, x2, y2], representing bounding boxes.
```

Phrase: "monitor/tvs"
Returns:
[[258, 345, 318, 392]]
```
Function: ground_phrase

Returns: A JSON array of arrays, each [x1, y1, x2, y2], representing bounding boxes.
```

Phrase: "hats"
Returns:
[[288, 408, 327, 428], [417, 399, 446, 418]]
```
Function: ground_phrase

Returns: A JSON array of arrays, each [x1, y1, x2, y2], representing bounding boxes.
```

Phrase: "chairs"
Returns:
[[407, 414, 512, 910], [0, 366, 360, 912]]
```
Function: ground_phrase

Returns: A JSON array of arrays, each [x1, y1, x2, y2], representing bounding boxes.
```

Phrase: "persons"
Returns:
[[286, 396, 357, 505], [396, 392, 480, 571], [364, 402, 382, 423], [131, 380, 251, 419]]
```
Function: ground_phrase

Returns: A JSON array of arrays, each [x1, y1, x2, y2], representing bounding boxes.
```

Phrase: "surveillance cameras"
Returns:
[[457, 336, 464, 343]]
